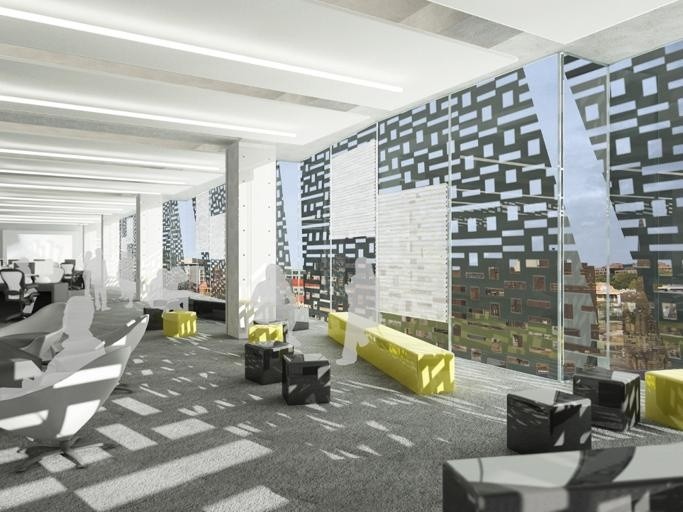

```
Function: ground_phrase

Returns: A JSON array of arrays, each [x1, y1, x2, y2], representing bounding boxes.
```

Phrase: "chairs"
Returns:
[[1, 301, 150, 473], [0, 258, 84, 322]]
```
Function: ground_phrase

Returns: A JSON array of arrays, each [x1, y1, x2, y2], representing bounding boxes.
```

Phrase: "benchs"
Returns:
[[189, 298, 226, 320], [442, 438, 683, 512], [328, 311, 454, 396]]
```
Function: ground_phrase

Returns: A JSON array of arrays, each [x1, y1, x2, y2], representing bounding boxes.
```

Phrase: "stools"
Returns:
[[143, 307, 198, 338], [243, 322, 331, 405], [506, 366, 682, 452]]
